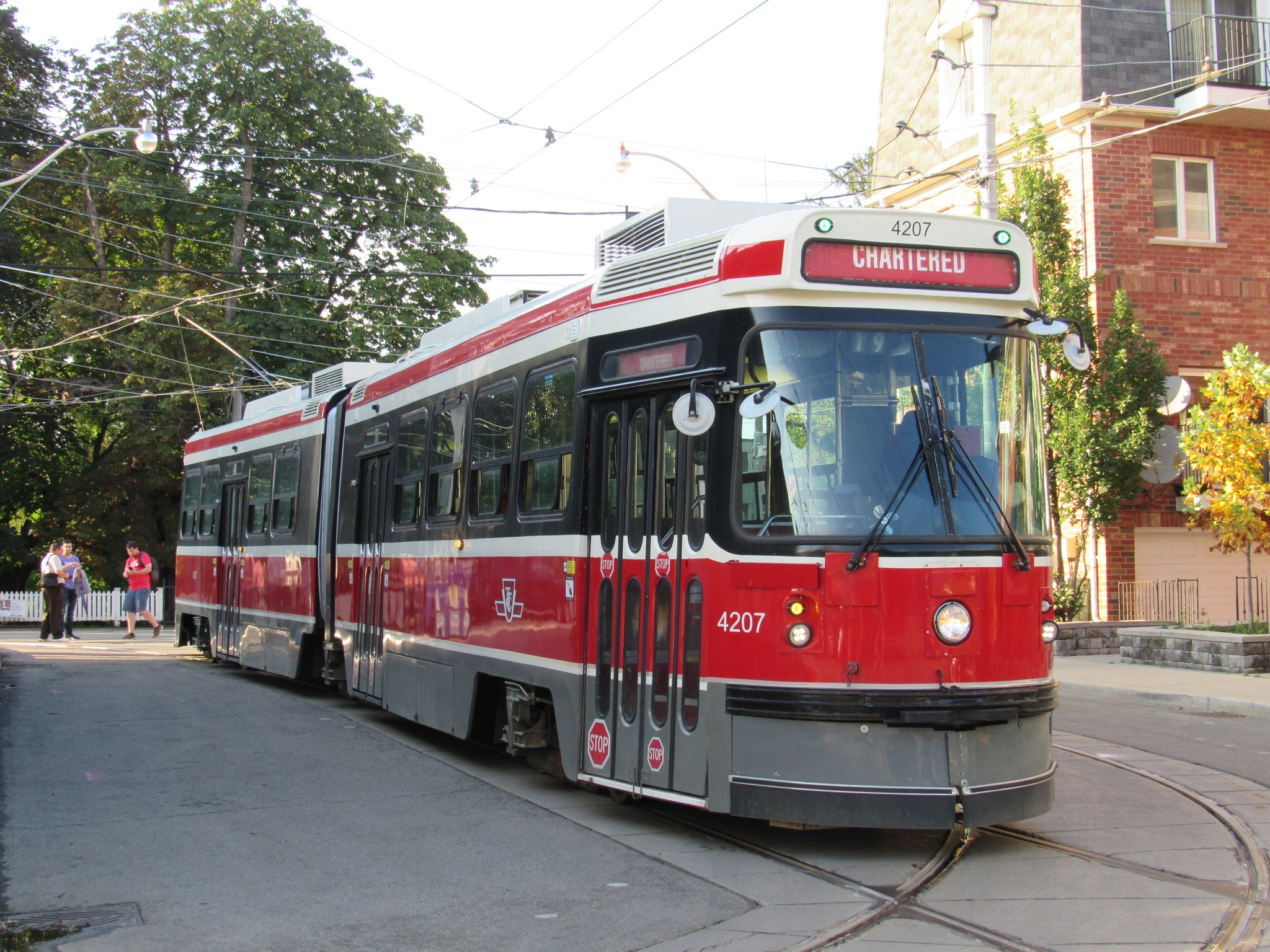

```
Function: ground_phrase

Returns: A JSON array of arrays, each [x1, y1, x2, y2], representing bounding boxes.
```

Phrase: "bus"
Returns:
[[174, 197, 1091, 834]]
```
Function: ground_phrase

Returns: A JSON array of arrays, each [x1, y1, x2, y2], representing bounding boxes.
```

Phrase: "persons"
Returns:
[[122, 542, 163, 640], [59, 539, 82, 639], [40, 543, 70, 642], [855, 409, 998, 516]]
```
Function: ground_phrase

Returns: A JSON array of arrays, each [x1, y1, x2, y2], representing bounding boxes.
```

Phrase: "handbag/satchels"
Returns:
[[41, 572, 58, 588]]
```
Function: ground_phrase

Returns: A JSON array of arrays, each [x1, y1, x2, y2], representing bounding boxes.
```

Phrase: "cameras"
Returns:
[[68, 575, 72, 579]]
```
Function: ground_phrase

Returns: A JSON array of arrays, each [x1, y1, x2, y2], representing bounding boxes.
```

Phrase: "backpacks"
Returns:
[[139, 551, 159, 583]]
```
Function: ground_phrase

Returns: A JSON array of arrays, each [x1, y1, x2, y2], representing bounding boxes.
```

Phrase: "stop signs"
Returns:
[[600, 552, 614, 578], [655, 552, 669, 577], [647, 737, 664, 771], [588, 719, 610, 770]]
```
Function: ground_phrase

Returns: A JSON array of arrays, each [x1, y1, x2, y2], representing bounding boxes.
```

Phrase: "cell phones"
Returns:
[[128, 567, 133, 570]]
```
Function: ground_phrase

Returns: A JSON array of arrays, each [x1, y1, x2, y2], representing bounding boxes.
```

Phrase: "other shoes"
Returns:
[[64, 633, 80, 640], [121, 632, 136, 640], [152, 624, 163, 639], [39, 637, 53, 642], [51, 637, 70, 643]]
